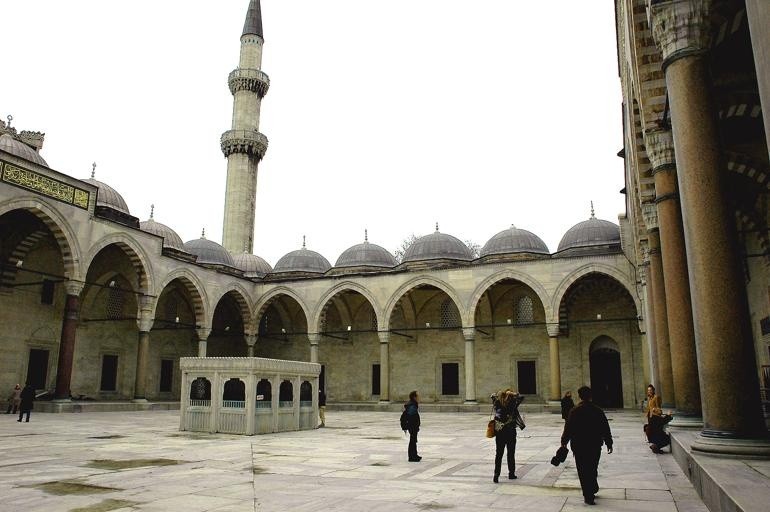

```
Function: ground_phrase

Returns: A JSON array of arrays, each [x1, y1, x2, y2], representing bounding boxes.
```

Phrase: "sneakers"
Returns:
[[650, 445, 664, 455], [319, 424, 324, 427], [594, 485, 599, 493], [12, 412, 16, 414], [26, 420, 29, 422], [584, 500, 595, 504], [4, 412, 10, 414], [409, 455, 422, 462], [493, 476, 498, 483], [17, 419, 22, 422], [509, 476, 517, 479]]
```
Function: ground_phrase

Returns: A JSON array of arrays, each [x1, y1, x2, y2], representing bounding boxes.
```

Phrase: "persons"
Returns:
[[404, 390, 423, 462], [644, 384, 662, 446], [491, 387, 527, 484], [561, 384, 615, 508], [5, 384, 21, 414], [318, 386, 327, 429], [646, 406, 675, 455], [16, 380, 37, 423], [560, 388, 575, 424]]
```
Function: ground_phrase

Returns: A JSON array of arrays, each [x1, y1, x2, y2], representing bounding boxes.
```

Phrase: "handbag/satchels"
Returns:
[[486, 420, 495, 438]]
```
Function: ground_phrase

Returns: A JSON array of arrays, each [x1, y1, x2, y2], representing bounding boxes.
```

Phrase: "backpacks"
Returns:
[[400, 405, 408, 434]]
